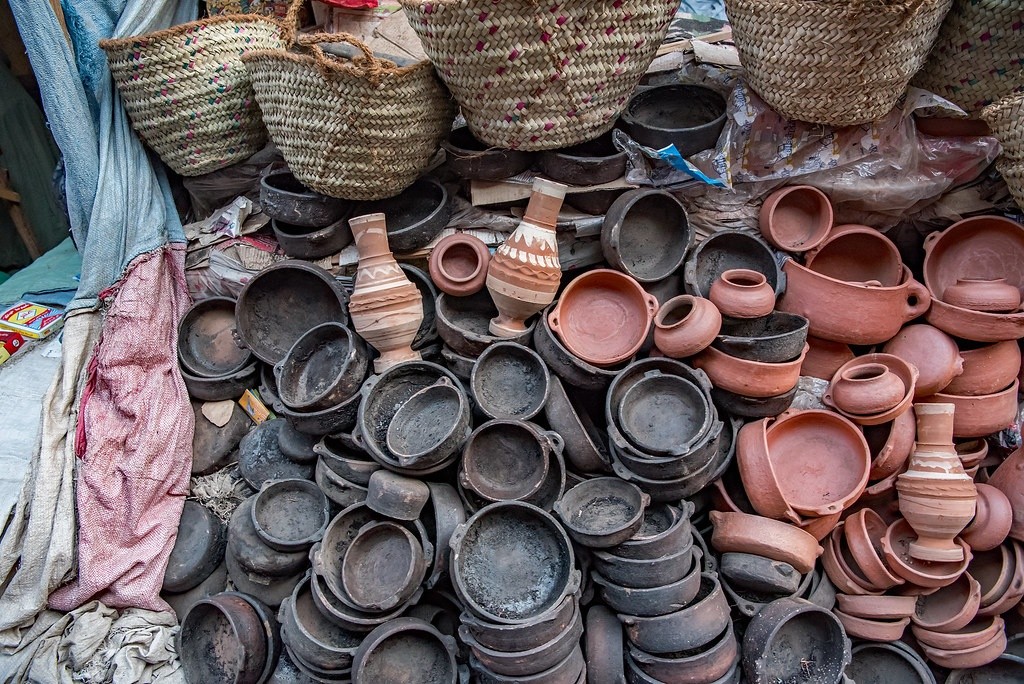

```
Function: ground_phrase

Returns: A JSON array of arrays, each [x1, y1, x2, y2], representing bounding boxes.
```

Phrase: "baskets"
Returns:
[[241, 0, 460, 201], [909, 0, 1024, 119], [723, 0, 955, 126], [99, 0, 284, 176], [398, 0, 682, 151], [981, 91, 1024, 212]]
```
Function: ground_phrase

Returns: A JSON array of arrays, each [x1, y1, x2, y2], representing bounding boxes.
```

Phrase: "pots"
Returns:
[[158, 83, 1024, 684]]
